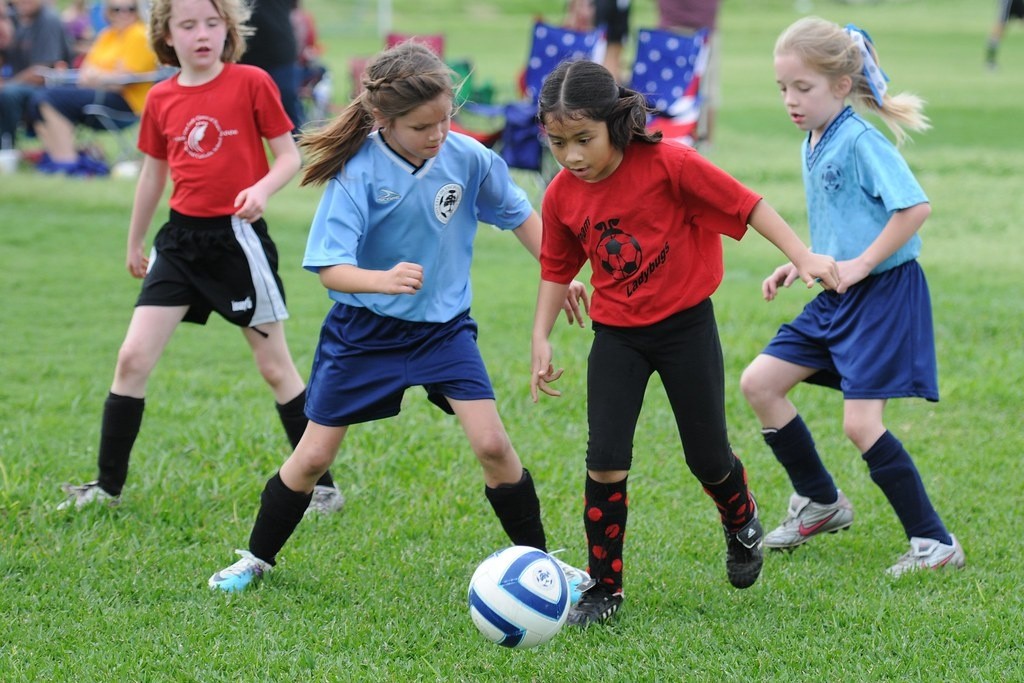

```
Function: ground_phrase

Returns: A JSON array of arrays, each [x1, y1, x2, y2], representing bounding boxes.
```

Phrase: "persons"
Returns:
[[591, 0, 630, 84], [43, 0, 346, 515], [740, 14, 967, 578], [0, 0, 164, 175], [236, 0, 311, 141], [207, 40, 597, 606], [295, 8, 325, 103], [530, 60, 840, 632], [985, 0, 1023, 66]]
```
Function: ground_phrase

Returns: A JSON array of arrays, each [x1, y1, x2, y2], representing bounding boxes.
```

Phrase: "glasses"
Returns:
[[107, 4, 137, 13]]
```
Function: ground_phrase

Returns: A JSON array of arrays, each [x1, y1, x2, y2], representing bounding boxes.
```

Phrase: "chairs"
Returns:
[[466, 18, 607, 171], [38, 64, 181, 177], [626, 26, 715, 139], [351, 33, 505, 149]]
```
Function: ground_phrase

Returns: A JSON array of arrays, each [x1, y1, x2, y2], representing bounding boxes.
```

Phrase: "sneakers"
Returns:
[[882, 534, 964, 583], [545, 550, 596, 606], [566, 579, 624, 629], [209, 548, 274, 593], [720, 490, 763, 590], [762, 490, 856, 550], [57, 481, 121, 511]]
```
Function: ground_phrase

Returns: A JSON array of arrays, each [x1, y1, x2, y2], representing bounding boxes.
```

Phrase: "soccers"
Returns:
[[467, 544, 572, 649]]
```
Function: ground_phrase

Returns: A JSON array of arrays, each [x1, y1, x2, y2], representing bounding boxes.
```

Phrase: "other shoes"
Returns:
[[305, 485, 345, 522]]
